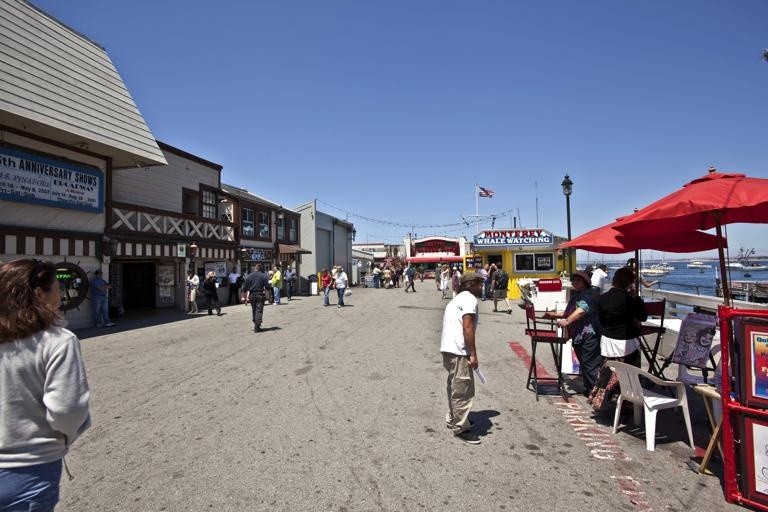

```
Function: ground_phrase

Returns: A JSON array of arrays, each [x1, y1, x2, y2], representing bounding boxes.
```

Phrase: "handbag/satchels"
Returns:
[[344, 287, 353, 296], [389, 279, 394, 285], [291, 278, 298, 287]]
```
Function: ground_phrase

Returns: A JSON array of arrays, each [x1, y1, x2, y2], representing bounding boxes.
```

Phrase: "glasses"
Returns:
[[572, 278, 581, 282]]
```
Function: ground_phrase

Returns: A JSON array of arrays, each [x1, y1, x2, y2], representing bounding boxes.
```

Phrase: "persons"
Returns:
[[271, 267, 282, 305], [333, 266, 350, 308], [626, 259, 659, 297], [1, 256, 91, 502], [320, 268, 333, 306], [370, 261, 424, 292], [186, 269, 200, 314], [583, 264, 593, 278], [91, 270, 116, 327], [440, 271, 485, 444], [203, 271, 222, 316], [478, 263, 490, 300], [267, 271, 274, 303], [284, 266, 297, 301], [246, 264, 269, 332], [588, 267, 648, 405], [591, 263, 608, 291], [490, 263, 512, 314], [434, 262, 462, 299], [227, 267, 241, 305], [545, 272, 602, 390], [331, 266, 337, 283]]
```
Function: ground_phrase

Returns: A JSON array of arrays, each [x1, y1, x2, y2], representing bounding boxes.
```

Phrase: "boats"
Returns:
[[608, 266, 623, 269], [739, 265, 768, 271]]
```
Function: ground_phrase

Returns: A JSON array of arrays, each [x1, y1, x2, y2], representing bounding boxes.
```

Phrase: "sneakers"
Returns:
[[336, 304, 341, 308], [454, 429, 482, 444], [468, 418, 474, 426], [273, 303, 280, 306], [341, 304, 344, 306], [188, 309, 194, 314], [96, 324, 101, 328], [507, 307, 513, 314], [323, 303, 329, 306], [492, 309, 498, 313], [104, 322, 116, 327], [194, 309, 199, 313]]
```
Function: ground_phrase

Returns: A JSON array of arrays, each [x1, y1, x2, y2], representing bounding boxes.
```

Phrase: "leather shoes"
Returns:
[[217, 312, 223, 316], [405, 289, 409, 293], [413, 290, 417, 292], [208, 311, 214, 315]]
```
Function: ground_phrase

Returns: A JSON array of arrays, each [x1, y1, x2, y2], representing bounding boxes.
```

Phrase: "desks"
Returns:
[[691, 385, 732, 473]]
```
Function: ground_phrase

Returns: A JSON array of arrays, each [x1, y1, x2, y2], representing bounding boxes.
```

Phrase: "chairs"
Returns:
[[636, 299, 666, 379], [606, 361, 694, 451], [521, 298, 568, 404]]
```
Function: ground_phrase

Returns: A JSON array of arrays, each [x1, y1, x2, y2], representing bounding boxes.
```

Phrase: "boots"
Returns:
[[287, 296, 289, 299], [441, 292, 444, 298], [254, 324, 264, 333], [443, 294, 446, 298], [288, 296, 291, 301]]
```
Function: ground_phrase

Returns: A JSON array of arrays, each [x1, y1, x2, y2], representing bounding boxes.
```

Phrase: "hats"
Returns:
[[460, 271, 485, 281], [95, 269, 104, 274], [453, 267, 457, 270], [573, 270, 592, 285]]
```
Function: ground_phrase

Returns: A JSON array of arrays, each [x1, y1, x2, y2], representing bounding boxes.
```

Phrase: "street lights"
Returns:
[[562, 175, 574, 282]]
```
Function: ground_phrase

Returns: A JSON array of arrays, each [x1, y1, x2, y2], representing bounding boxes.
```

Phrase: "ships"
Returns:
[[650, 265, 676, 270], [638, 269, 664, 277], [725, 262, 743, 268], [686, 261, 712, 269]]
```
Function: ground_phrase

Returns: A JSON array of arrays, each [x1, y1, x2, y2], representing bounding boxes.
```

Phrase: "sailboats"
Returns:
[[593, 261, 597, 269], [641, 260, 648, 268], [576, 267, 586, 271], [659, 255, 667, 267]]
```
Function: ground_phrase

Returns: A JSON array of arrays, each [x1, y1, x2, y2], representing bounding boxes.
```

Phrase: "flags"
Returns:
[[479, 186, 496, 199]]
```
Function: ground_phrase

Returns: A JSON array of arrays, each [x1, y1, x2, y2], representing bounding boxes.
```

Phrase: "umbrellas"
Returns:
[[555, 207, 728, 295], [612, 166, 767, 308]]
